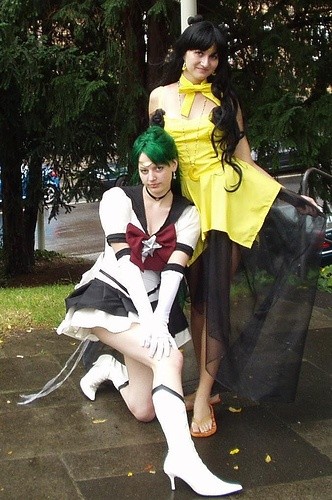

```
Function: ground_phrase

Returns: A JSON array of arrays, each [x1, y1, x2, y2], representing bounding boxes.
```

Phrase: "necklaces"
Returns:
[[177, 81, 207, 181]]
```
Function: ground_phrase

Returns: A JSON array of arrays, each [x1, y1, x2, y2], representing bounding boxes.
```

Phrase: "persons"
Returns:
[[56, 126, 242, 496], [147, 15, 328, 437]]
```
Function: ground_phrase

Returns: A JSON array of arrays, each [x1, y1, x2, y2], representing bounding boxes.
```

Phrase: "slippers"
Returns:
[[191, 402, 217, 437], [184, 393, 220, 411]]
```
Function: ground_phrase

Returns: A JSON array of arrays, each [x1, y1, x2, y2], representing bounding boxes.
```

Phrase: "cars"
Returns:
[[88, 157, 133, 187], [250, 142, 307, 174], [0, 163, 60, 204]]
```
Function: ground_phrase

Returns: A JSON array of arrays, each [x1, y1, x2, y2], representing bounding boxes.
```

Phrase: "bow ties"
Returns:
[[176, 74, 222, 117], [123, 224, 177, 272]]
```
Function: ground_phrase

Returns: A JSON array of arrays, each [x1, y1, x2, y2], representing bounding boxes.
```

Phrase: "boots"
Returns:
[[151, 384, 243, 497], [79, 353, 130, 401]]
